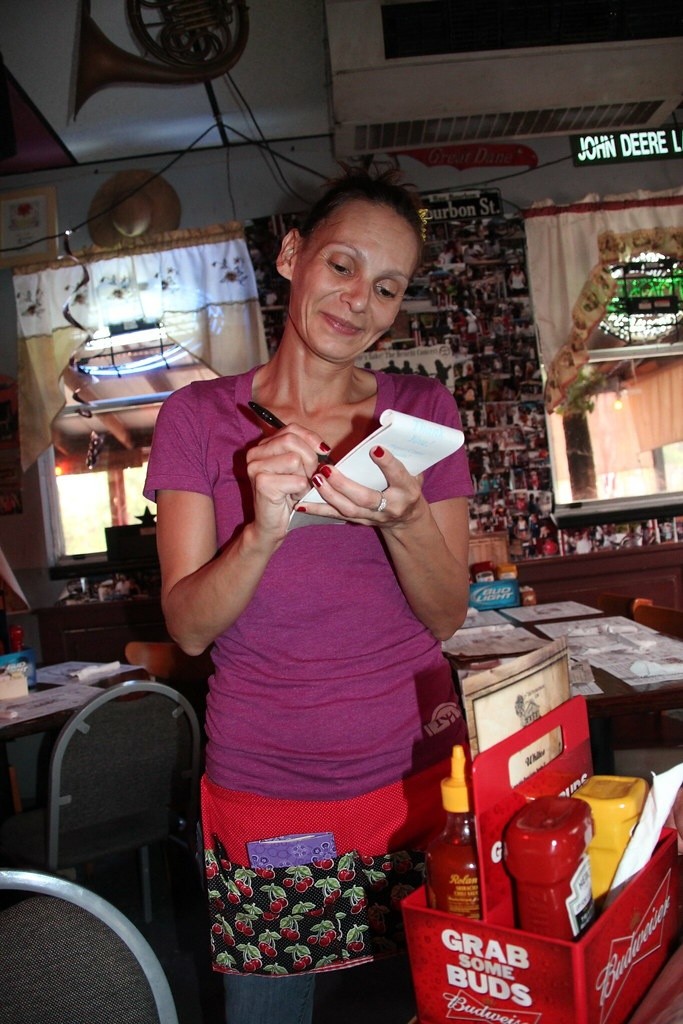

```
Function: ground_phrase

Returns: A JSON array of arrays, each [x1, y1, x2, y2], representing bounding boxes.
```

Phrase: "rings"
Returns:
[[378, 491, 387, 512]]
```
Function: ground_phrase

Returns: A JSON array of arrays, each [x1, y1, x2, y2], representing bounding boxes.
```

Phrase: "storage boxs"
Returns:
[[398, 825, 682, 1023]]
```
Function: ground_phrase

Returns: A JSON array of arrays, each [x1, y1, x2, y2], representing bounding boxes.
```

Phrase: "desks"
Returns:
[[35, 597, 214, 690], [440, 600, 683, 751]]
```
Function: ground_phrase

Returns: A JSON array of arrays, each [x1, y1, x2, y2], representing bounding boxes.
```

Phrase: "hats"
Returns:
[[86, 168, 181, 247]]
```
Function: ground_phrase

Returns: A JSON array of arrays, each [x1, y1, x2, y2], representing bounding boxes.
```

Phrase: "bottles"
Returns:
[[428, 744, 481, 920]]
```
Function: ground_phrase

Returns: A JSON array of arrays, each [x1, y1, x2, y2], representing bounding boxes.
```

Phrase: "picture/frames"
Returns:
[[0, 181, 60, 268]]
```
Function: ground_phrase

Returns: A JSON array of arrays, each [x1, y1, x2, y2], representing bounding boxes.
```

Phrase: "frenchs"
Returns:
[[72, 1, 251, 125]]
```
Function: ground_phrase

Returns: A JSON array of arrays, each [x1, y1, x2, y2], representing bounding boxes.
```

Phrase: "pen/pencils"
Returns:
[[248, 400, 336, 467]]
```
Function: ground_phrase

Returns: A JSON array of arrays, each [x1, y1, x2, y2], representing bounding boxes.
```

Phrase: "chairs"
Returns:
[[0, 685, 208, 1024]]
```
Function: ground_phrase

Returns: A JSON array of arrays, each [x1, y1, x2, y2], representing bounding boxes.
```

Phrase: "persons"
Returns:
[[143, 159, 476, 1024]]
